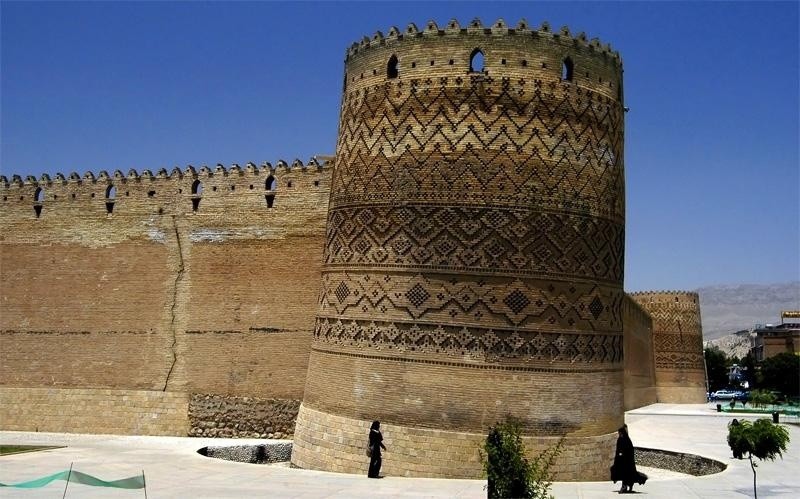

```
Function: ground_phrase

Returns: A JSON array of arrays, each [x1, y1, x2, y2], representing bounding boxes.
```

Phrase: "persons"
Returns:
[[366, 420, 387, 479], [613, 426, 637, 494]]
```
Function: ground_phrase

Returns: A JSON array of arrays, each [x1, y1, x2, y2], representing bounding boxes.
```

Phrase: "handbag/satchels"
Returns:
[[366, 446, 372, 457]]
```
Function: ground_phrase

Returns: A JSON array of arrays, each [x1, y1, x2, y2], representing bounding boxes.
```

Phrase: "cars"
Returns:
[[710, 390, 750, 401]]
